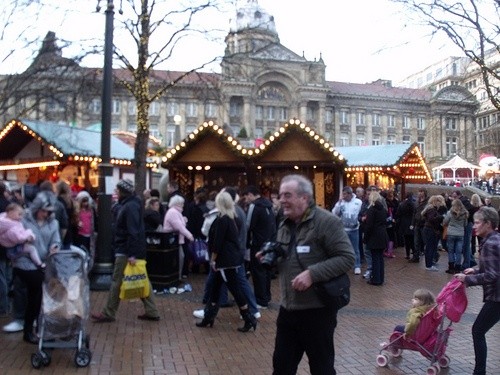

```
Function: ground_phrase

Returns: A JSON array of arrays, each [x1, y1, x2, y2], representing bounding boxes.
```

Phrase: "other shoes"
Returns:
[[408, 258, 419, 263], [192, 308, 205, 318], [354, 267, 362, 275], [363, 270, 373, 280], [366, 277, 382, 285], [3, 320, 23, 332], [22, 327, 40, 344], [425, 265, 439, 271], [137, 313, 161, 321], [91, 311, 114, 322]]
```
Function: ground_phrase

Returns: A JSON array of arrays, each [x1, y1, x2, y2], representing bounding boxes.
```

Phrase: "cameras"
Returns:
[[258, 241, 287, 270]]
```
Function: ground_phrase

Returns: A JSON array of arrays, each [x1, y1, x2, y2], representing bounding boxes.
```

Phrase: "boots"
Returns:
[[195, 304, 218, 328], [444, 262, 455, 274], [454, 264, 461, 274], [236, 307, 257, 333]]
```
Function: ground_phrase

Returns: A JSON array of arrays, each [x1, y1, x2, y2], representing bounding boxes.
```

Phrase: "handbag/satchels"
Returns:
[[313, 269, 351, 311], [119, 259, 150, 301]]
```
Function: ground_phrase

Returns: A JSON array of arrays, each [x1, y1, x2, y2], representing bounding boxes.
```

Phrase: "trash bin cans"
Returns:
[[145, 230, 179, 292]]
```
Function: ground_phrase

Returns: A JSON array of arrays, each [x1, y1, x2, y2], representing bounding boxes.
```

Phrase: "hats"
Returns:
[[40, 200, 60, 211], [117, 178, 134, 197]]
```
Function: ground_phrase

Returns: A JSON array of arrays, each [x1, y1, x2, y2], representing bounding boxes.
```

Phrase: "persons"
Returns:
[[380, 289, 435, 346], [89, 179, 160, 321], [0, 178, 94, 342], [453, 206, 500, 375], [355, 187, 491, 277], [441, 180, 500, 195], [255, 174, 355, 375], [143, 186, 285, 332], [332, 186, 363, 275], [363, 191, 388, 285]]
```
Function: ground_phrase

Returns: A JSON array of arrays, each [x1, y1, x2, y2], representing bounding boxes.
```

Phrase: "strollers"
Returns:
[[375, 269, 467, 375], [29, 243, 93, 367]]
